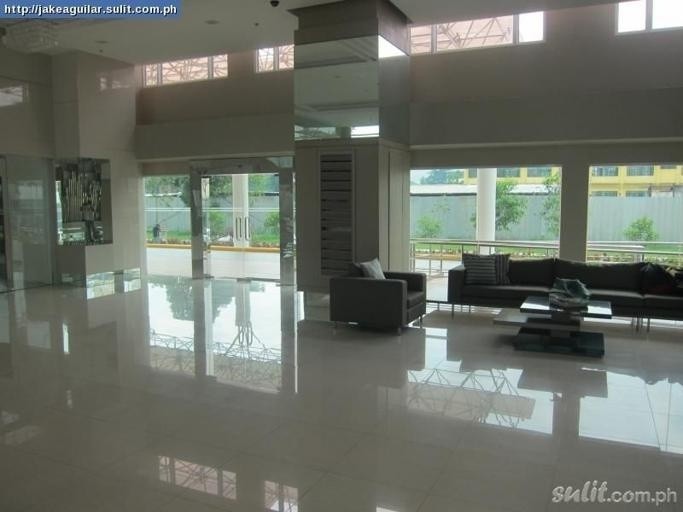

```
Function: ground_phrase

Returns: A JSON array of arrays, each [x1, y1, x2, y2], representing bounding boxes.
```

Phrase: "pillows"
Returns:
[[461, 252, 679, 293], [360, 257, 386, 279]]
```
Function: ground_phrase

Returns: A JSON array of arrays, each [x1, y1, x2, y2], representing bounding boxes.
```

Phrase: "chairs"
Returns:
[[330, 267, 426, 330]]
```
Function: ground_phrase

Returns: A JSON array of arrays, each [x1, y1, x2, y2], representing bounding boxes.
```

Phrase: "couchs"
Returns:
[[447, 265, 683, 333]]
